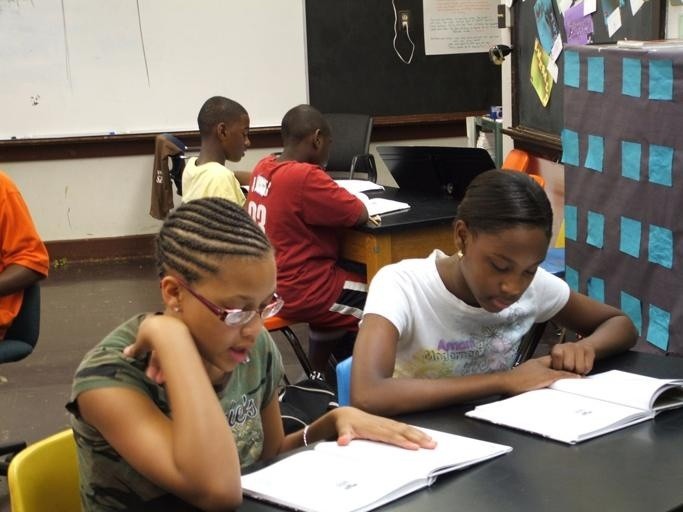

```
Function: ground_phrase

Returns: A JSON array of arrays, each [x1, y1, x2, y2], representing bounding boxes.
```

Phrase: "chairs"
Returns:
[[528, 171, 545, 189], [319, 110, 381, 183], [336, 355, 355, 405], [0, 279, 43, 477], [5, 429, 82, 512], [155, 131, 248, 202], [498, 147, 528, 174]]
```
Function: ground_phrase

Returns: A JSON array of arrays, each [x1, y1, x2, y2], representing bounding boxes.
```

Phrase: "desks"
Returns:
[[238, 178, 462, 292], [136, 349, 683, 512]]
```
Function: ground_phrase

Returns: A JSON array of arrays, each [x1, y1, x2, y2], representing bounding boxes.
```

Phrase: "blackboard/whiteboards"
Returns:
[[1, 1, 504, 149]]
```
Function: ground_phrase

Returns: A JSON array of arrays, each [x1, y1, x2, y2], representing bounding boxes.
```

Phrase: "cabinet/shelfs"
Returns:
[[465, 111, 502, 169]]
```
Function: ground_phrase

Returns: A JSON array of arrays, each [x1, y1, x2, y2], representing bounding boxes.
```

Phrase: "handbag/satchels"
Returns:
[[278, 379, 340, 435]]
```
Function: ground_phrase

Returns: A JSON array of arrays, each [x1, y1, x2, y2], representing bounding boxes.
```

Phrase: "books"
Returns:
[[331, 177, 385, 194], [237, 421, 512, 512], [0, 171, 50, 349], [464, 364, 683, 447], [348, 190, 411, 217]]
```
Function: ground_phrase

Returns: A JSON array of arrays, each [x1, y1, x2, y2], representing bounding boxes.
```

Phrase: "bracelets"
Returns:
[[300, 423, 310, 447]]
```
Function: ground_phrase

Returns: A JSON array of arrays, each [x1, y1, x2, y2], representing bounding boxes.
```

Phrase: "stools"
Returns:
[[261, 313, 316, 379]]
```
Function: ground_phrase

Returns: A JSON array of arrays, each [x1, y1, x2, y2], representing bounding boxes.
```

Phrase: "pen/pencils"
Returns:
[[368, 217, 379, 226]]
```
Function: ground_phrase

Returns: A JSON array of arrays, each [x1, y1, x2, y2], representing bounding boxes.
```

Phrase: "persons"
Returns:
[[242, 102, 372, 384], [62, 192, 441, 512], [180, 93, 249, 210], [347, 165, 640, 422]]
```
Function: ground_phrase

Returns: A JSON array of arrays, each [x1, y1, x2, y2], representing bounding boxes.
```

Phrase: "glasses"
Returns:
[[160, 274, 284, 329]]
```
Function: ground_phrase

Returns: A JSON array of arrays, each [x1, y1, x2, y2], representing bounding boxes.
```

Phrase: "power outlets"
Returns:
[[398, 9, 407, 30]]
